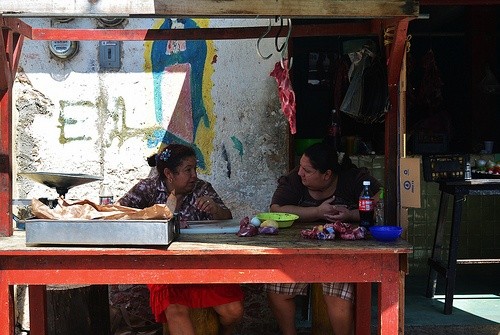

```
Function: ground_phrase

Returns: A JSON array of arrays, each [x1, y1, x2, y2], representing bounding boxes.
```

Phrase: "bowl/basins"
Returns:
[[370, 225, 403, 243], [294, 138, 322, 154], [256, 212, 299, 228]]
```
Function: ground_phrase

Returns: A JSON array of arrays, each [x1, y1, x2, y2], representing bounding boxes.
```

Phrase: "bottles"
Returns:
[[359, 180, 376, 232], [374, 187, 384, 225]]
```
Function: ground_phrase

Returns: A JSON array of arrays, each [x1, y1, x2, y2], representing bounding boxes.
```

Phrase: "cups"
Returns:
[[485, 141, 493, 153]]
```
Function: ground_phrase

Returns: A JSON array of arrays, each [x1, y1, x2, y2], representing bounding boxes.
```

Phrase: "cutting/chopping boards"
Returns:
[[177, 218, 242, 234]]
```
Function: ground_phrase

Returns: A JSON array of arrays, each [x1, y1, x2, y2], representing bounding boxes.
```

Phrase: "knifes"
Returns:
[[187, 222, 220, 225]]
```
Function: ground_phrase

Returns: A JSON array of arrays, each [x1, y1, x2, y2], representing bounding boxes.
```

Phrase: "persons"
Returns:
[[115, 145, 244, 335], [265, 143, 380, 335]]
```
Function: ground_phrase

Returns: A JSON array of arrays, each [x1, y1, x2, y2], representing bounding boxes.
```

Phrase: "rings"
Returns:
[[207, 201, 210, 202]]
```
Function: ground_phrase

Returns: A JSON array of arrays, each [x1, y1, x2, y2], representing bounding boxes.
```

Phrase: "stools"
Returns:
[[162, 308, 218, 335], [313, 283, 354, 335]]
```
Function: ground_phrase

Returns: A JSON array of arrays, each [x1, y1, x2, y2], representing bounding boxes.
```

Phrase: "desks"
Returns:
[[425, 185, 500, 314], [0, 222, 414, 335]]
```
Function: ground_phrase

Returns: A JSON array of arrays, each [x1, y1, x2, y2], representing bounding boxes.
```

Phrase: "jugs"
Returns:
[[474, 156, 487, 167], [486, 160, 496, 168]]
